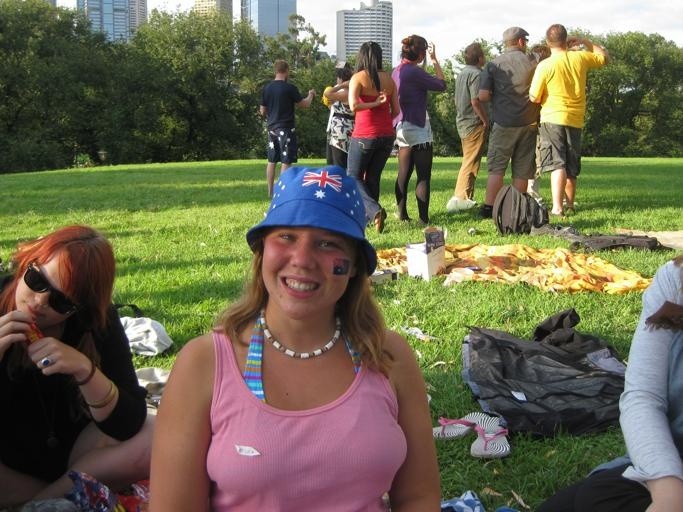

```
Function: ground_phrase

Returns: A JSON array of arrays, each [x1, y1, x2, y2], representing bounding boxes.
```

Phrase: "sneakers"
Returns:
[[375, 208, 387, 233], [446, 195, 476, 211]]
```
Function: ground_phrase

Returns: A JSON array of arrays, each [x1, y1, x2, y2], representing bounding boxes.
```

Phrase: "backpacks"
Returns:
[[460, 307, 628, 437], [493, 183, 538, 236]]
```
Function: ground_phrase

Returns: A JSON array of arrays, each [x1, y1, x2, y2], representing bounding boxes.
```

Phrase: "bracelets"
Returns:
[[76, 361, 96, 386], [86, 380, 117, 409]]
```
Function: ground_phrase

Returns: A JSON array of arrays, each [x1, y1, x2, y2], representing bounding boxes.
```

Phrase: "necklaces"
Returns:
[[260, 307, 342, 359], [376, 71, 385, 72]]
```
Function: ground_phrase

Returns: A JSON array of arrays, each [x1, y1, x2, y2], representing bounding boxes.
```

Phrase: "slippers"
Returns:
[[434, 412, 513, 459]]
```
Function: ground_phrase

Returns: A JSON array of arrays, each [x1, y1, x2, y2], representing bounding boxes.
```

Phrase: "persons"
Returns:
[[536, 251, 683, 511], [446, 43, 489, 212], [324, 61, 355, 171], [529, 25, 607, 216], [0, 224, 156, 512], [149, 161, 441, 512], [391, 35, 446, 224], [479, 27, 540, 219], [259, 60, 316, 198], [346, 41, 400, 231]]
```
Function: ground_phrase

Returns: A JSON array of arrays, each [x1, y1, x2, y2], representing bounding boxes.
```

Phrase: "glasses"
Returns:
[[23, 261, 78, 315]]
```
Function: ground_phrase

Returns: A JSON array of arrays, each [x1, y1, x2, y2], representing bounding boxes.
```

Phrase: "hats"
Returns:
[[246, 164, 376, 277], [502, 26, 530, 41]]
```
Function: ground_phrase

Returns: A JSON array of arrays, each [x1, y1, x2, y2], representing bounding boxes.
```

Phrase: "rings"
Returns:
[[42, 357, 51, 367]]
